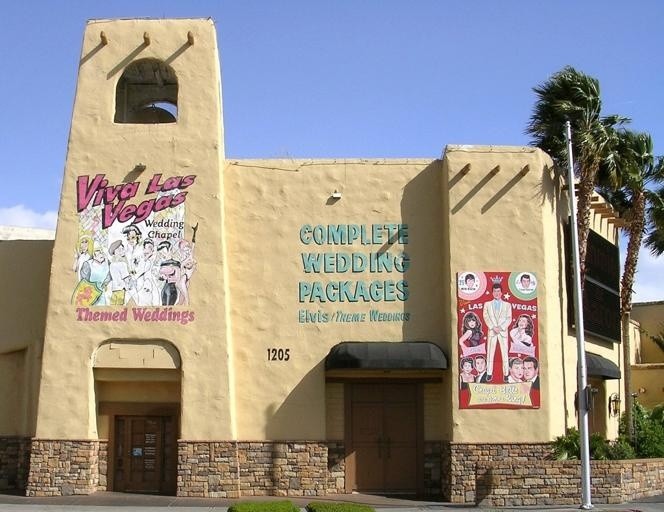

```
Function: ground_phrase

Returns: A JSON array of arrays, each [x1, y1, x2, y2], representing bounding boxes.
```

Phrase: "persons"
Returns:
[[457, 273, 539, 390], [74, 220, 200, 307]]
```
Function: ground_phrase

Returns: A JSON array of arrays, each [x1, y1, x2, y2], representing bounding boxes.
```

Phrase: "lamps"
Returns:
[[613, 394, 621, 414]]
